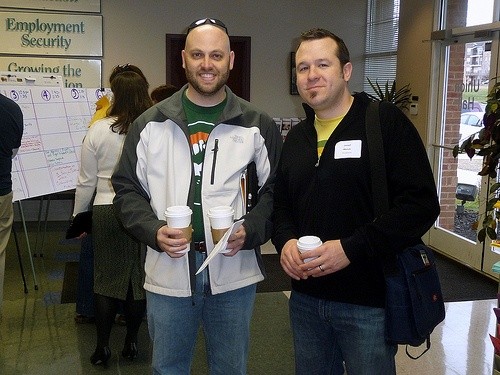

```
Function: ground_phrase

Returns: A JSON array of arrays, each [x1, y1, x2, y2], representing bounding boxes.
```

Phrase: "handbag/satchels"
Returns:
[[384, 241, 445, 347]]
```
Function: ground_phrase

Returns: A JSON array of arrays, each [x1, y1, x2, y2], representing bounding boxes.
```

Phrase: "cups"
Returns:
[[164, 205, 193, 254], [206, 206, 236, 254], [296, 236, 323, 271]]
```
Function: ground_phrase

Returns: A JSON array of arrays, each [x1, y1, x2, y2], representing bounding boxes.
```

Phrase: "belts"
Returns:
[[194, 241, 207, 253]]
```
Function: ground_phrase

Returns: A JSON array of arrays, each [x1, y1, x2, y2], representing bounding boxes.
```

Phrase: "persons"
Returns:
[[151, 84, 180, 104], [66, 71, 155, 370], [270, 28, 441, 375], [110, 17, 283, 375], [75, 64, 150, 327], [0, 93, 23, 324]]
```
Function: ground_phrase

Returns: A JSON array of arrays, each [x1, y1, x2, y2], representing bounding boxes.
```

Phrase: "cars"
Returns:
[[460, 101, 488, 152]]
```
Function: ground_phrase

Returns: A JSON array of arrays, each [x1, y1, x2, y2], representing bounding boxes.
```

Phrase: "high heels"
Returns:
[[121, 343, 137, 361], [90, 346, 111, 368]]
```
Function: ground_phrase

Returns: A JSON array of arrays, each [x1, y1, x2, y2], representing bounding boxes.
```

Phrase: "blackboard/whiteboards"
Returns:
[[0, 86, 116, 203]]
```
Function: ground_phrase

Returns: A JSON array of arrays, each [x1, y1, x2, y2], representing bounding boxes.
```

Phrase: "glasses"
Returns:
[[187, 17, 229, 35]]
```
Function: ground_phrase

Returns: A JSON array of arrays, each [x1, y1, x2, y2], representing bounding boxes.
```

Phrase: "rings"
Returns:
[[319, 265, 325, 272]]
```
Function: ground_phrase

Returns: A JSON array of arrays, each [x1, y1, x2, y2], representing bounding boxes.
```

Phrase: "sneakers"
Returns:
[[74, 313, 96, 324], [114, 313, 127, 325]]
